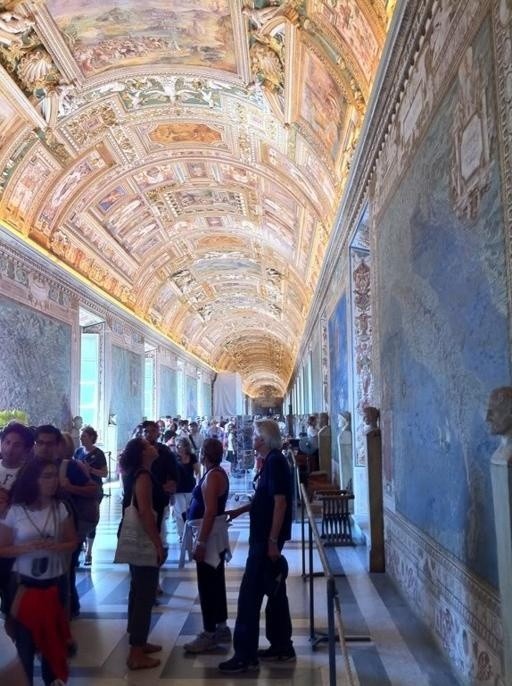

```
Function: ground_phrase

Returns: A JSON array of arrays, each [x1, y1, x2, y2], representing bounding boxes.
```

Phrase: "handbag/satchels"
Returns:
[[112, 506, 160, 567]]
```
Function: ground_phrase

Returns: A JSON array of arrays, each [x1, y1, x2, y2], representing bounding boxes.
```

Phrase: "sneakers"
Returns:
[[184, 625, 232, 652], [218, 655, 260, 675], [258, 644, 297, 663]]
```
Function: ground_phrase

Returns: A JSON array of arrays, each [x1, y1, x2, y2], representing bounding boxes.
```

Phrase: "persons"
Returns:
[[361, 407, 379, 430], [337, 411, 351, 446], [481, 386, 511, 441]]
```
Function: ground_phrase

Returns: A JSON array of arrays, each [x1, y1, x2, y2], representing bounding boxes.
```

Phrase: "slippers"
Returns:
[[127, 643, 161, 670]]
